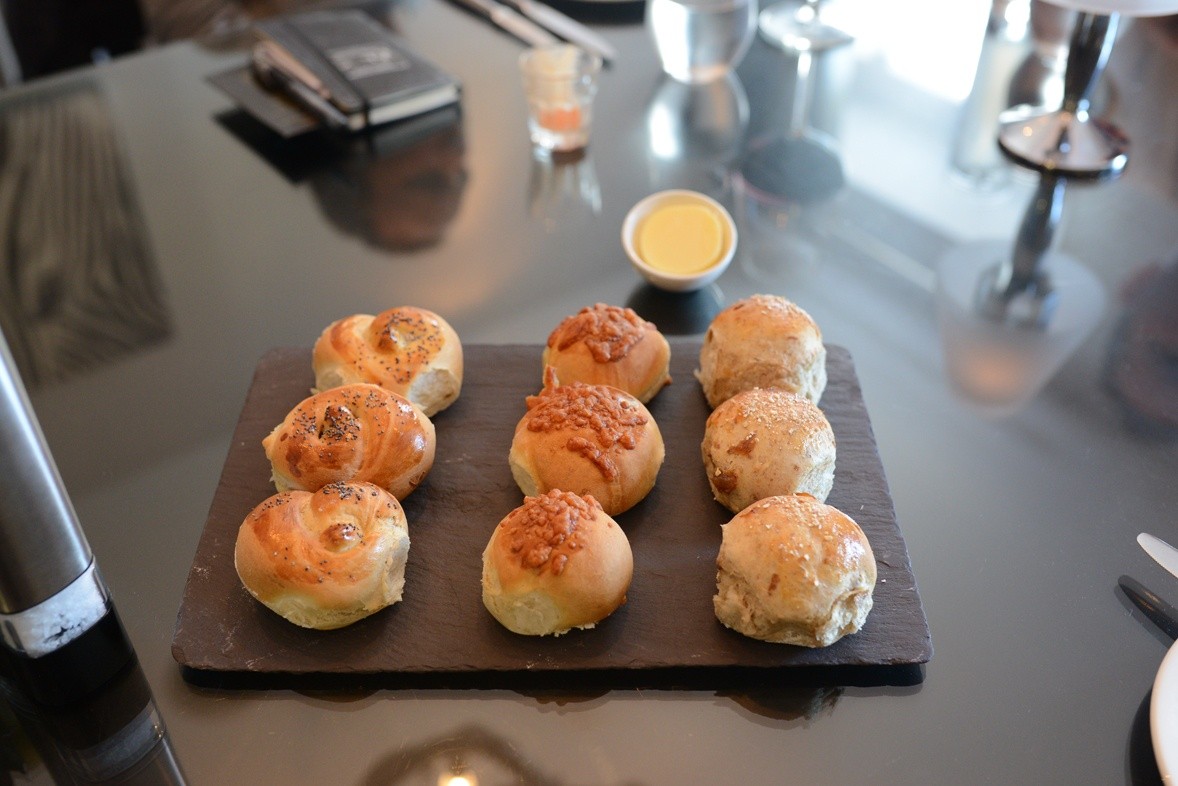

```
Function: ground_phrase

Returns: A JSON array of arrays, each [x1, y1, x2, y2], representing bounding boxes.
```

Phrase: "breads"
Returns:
[[259, 383, 436, 501], [234, 477, 414, 632], [309, 305, 464, 418], [509, 380, 665, 518], [701, 387, 838, 514], [480, 488, 634, 638], [713, 494, 877, 650], [541, 302, 671, 406], [693, 293, 830, 409]]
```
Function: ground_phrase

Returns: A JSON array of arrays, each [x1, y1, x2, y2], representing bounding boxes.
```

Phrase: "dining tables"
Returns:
[[4, 1, 1178, 786]]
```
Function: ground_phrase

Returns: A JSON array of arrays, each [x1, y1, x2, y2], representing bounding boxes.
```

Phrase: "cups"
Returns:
[[643, 0, 760, 82], [519, 37, 602, 150]]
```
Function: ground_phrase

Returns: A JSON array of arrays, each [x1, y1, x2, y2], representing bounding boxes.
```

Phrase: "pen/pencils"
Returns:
[[249, 59, 347, 130]]
